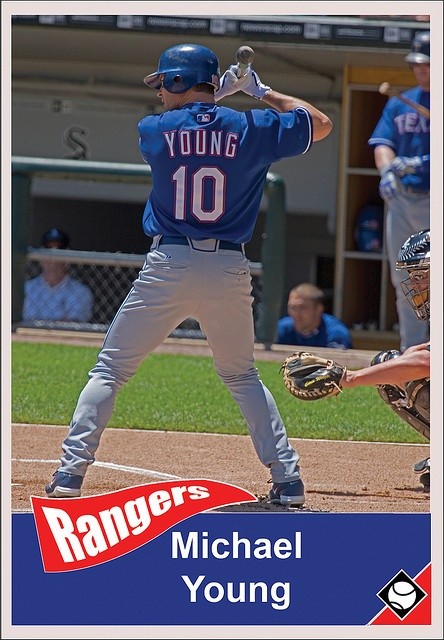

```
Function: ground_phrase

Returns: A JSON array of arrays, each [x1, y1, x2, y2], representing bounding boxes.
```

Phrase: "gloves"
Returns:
[[214, 67, 252, 103], [379, 165, 401, 201], [228, 64, 272, 101], [391, 155, 422, 177]]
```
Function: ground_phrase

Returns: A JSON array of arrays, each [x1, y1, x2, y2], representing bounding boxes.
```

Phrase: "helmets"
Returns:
[[404, 31, 430, 63], [144, 44, 219, 93], [396, 228, 430, 322]]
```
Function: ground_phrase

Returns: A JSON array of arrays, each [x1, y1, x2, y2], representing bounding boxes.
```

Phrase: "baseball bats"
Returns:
[[235, 45, 256, 77], [379, 82, 429, 119]]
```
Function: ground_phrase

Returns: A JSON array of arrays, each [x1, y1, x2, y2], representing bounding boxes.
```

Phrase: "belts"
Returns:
[[158, 236, 242, 252]]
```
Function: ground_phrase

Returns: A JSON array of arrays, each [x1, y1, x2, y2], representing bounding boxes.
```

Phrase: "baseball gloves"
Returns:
[[280, 352, 346, 401]]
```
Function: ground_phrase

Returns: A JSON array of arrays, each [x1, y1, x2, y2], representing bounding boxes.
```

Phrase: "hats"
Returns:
[[43, 226, 68, 246]]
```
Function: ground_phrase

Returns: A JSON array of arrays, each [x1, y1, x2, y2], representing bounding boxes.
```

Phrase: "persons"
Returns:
[[367, 32, 430, 354], [21, 226, 94, 324], [275, 283, 352, 351], [280, 229, 429, 492], [45, 43, 332, 507]]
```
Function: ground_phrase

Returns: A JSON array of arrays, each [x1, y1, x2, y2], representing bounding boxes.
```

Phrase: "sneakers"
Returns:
[[45, 470, 83, 497], [267, 478, 305, 506]]
